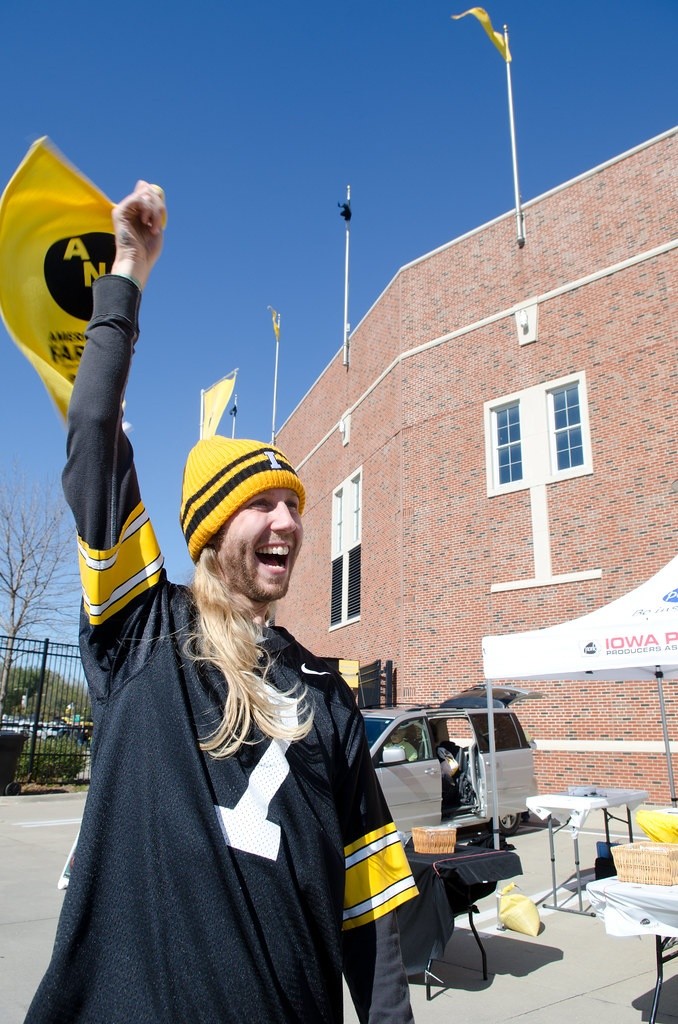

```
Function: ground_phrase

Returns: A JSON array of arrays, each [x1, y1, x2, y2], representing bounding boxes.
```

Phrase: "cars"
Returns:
[[2, 714, 93, 745]]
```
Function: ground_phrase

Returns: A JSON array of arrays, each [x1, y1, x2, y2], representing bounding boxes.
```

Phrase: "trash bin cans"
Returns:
[[0, 730, 30, 797]]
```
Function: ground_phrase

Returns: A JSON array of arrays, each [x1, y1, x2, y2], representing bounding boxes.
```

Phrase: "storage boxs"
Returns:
[[610, 841, 676, 884], [410, 826, 456, 855]]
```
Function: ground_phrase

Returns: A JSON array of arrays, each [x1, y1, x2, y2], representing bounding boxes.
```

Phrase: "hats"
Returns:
[[180, 435, 305, 563]]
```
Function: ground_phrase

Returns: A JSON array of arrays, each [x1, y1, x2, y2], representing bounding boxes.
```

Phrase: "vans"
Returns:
[[357, 683, 544, 844]]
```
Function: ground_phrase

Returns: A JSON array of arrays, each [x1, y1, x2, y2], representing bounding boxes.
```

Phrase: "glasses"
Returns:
[[390, 733, 398, 735]]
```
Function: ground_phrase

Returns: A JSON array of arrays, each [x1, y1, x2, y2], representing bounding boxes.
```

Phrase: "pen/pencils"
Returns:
[[584, 794, 602, 797]]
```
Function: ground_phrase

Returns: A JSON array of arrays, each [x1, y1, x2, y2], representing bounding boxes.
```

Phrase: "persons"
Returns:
[[383, 728, 418, 762], [20, 178, 423, 1023]]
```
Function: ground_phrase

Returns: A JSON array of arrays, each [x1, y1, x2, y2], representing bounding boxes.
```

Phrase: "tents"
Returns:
[[483, 549, 678, 932]]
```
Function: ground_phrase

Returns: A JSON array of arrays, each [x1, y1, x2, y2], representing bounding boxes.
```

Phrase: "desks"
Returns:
[[391, 837, 522, 1003], [521, 789, 649, 917], [584, 871, 678, 1023]]
